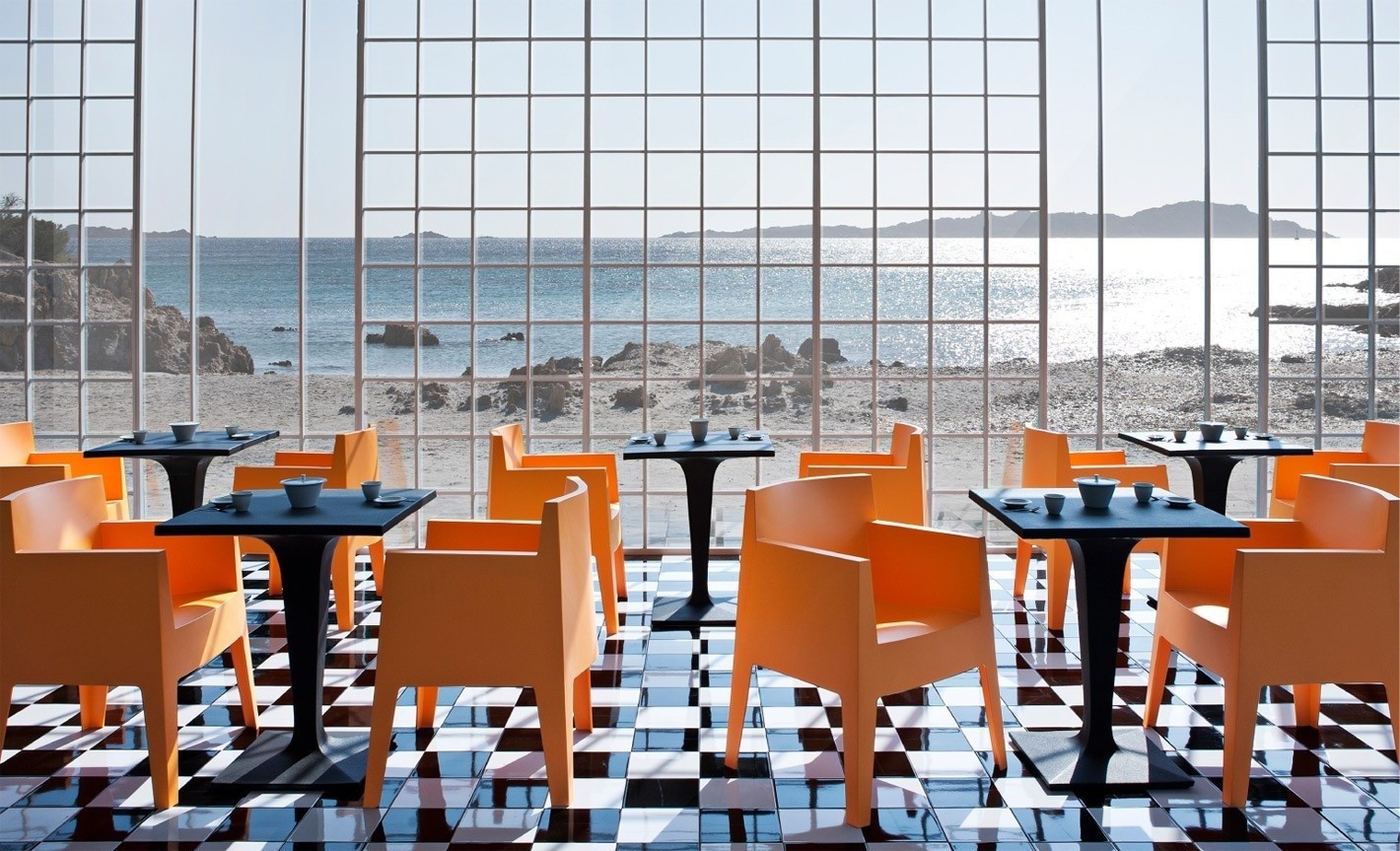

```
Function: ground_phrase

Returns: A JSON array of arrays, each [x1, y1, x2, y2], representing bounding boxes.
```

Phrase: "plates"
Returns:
[[631, 436, 652, 444], [999, 498, 1032, 508], [1255, 434, 1274, 440], [1163, 496, 1196, 506], [744, 434, 764, 440], [209, 497, 232, 509], [1148, 436, 1164, 441], [235, 433, 254, 439], [373, 496, 406, 505], [119, 435, 134, 443]]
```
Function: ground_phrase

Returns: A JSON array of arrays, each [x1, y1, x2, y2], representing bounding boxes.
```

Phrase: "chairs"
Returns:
[[800, 422, 927, 527], [231, 428, 385, 629], [0, 474, 260, 809], [1142, 473, 1400, 807], [362, 476, 601, 810], [723, 474, 1008, 826], [1012, 425, 1170, 631], [0, 421, 129, 519], [1267, 420, 1400, 518], [485, 421, 629, 636]]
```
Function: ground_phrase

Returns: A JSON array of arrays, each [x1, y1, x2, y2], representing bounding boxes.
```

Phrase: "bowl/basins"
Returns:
[[1198, 421, 1228, 441], [169, 422, 200, 441], [1073, 476, 1121, 508], [278, 474, 328, 509]]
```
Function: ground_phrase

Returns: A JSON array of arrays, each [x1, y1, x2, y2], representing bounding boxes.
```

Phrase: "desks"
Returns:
[[966, 488, 1251, 790], [623, 431, 776, 627], [154, 489, 438, 788], [84, 430, 280, 603], [1118, 431, 1315, 604]]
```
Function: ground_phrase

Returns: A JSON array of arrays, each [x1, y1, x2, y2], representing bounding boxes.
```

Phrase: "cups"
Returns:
[[1234, 426, 1249, 440], [360, 480, 382, 501], [1131, 482, 1155, 502], [654, 433, 666, 446], [133, 431, 147, 444], [1173, 430, 1186, 443], [690, 420, 709, 442], [230, 491, 254, 511], [1044, 494, 1065, 514], [224, 425, 239, 438], [728, 428, 741, 440]]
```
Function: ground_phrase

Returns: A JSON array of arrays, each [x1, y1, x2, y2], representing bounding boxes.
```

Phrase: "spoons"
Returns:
[[1004, 506, 1043, 512]]
